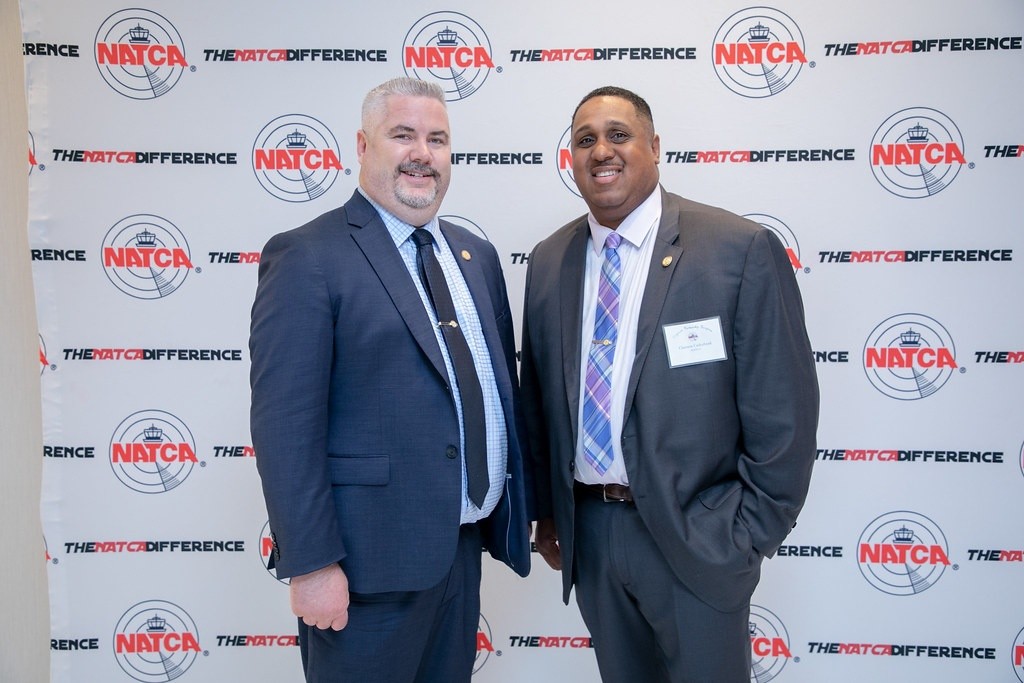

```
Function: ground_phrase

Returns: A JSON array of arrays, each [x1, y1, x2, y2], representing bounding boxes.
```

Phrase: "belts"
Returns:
[[573, 479, 633, 503]]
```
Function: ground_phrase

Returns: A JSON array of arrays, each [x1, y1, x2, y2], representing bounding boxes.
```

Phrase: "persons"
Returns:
[[519, 86, 820, 683], [248, 77, 533, 683]]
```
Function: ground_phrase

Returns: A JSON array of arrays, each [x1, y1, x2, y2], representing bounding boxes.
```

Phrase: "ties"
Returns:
[[411, 226, 491, 511], [582, 232, 622, 477]]
